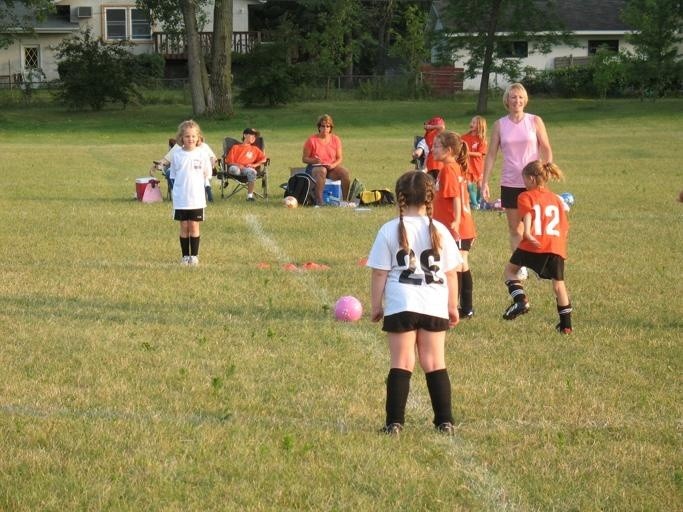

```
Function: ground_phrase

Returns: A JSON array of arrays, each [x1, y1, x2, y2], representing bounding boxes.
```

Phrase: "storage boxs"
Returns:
[[322, 178, 343, 205], [135, 177, 160, 202]]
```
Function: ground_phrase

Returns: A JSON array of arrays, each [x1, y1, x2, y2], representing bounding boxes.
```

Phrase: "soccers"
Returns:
[[561, 193, 575, 208], [335, 296, 362, 321], [283, 196, 298, 209]]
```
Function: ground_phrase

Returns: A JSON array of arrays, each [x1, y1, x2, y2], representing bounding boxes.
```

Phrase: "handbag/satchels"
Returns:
[[358, 188, 393, 207], [284, 173, 316, 207]]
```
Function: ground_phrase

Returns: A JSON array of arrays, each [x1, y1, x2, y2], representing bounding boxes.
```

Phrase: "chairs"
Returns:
[[217, 136, 271, 203], [412, 136, 424, 171], [153, 139, 176, 200]]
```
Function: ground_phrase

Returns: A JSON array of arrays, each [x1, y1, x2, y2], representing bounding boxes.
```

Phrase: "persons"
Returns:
[[431, 132, 476, 318], [483, 82, 553, 279], [364, 171, 464, 438], [502, 160, 573, 335], [410, 114, 445, 172], [145, 114, 351, 266], [460, 115, 488, 210]]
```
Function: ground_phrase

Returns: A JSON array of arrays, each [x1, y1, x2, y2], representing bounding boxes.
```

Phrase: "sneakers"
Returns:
[[380, 422, 401, 433], [245, 198, 254, 201], [559, 326, 573, 336], [437, 423, 453, 432], [460, 307, 473, 317], [181, 254, 197, 265], [504, 303, 529, 321]]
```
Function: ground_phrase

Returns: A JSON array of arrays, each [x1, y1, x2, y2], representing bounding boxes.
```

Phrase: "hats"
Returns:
[[243, 127, 258, 135]]
